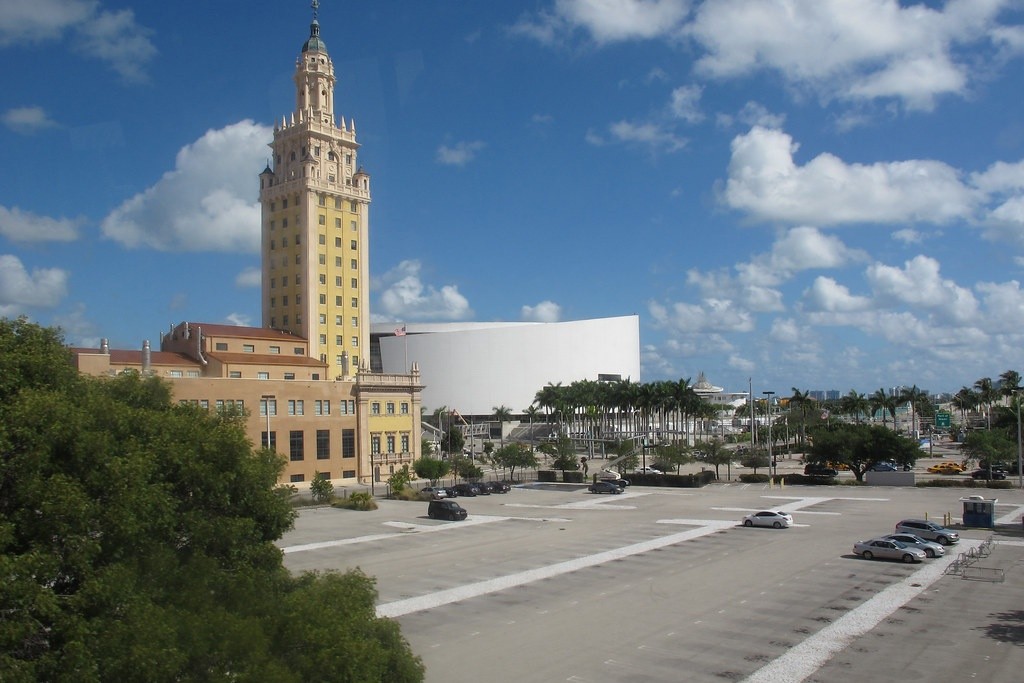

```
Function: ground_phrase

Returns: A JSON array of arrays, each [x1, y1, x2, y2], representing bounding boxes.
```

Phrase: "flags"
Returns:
[[393, 326, 405, 336]]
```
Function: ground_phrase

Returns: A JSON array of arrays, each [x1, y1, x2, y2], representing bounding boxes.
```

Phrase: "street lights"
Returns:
[[632, 409, 640, 451], [261, 394, 275, 451], [763, 391, 776, 482], [557, 409, 563, 457], [1011, 390, 1022, 484]]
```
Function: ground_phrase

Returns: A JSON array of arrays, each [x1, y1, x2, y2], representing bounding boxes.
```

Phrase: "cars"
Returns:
[[588, 481, 624, 494], [971, 459, 1008, 480], [634, 466, 661, 476], [486, 481, 512, 494], [824, 459, 912, 472], [881, 533, 946, 558], [470, 482, 495, 495], [441, 487, 458, 498], [927, 461, 967, 474], [452, 484, 480, 497], [418, 487, 446, 500], [742, 510, 793, 529], [852, 538, 927, 563]]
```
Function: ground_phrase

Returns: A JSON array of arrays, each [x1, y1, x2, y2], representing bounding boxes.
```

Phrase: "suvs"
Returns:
[[896, 518, 960, 545], [803, 464, 838, 478], [428, 500, 467, 520]]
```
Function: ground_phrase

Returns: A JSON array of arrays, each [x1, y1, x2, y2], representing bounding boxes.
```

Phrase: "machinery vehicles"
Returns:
[[593, 443, 645, 488]]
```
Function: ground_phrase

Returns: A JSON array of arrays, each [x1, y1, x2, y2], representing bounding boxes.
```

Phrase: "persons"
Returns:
[[580, 459, 588, 477]]
